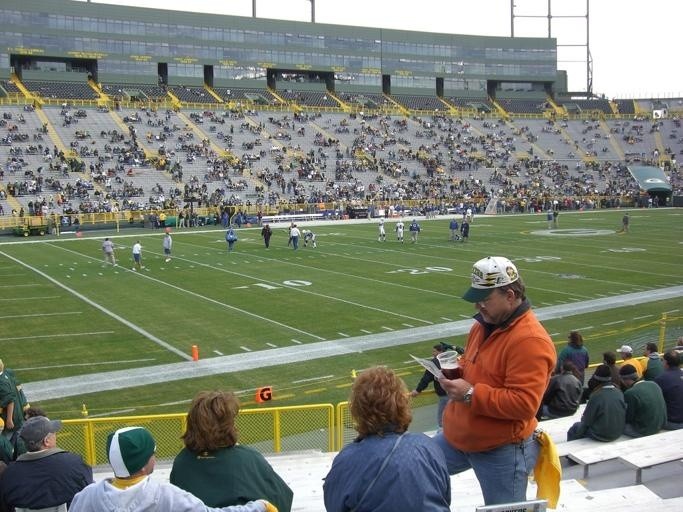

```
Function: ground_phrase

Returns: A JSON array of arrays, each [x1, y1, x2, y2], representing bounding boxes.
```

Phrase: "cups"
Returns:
[[436, 350, 459, 379]]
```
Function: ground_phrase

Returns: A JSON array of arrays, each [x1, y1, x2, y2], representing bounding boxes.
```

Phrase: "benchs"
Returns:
[[92, 403, 683, 512]]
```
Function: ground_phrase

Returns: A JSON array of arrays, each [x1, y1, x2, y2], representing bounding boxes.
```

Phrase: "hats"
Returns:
[[594, 365, 612, 380], [106, 426, 155, 478], [19, 416, 61, 445], [619, 365, 638, 379], [461, 256, 519, 304], [616, 345, 633, 354]]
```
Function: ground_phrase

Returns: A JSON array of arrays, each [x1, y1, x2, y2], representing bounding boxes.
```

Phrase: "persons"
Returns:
[[461, 218, 470, 242], [408, 341, 466, 429], [448, 217, 459, 241], [0, 81, 683, 237], [130, 240, 143, 272], [166, 390, 294, 512], [430, 256, 559, 510], [0, 416, 94, 511], [261, 224, 272, 250], [161, 233, 173, 262], [409, 219, 420, 244], [288, 223, 294, 245], [0, 359, 44, 472], [64, 425, 280, 511], [225, 224, 236, 253], [101, 238, 120, 271], [394, 219, 404, 245], [378, 217, 386, 242], [289, 225, 301, 249], [302, 228, 317, 247], [324, 366, 449, 512], [539, 328, 682, 466]]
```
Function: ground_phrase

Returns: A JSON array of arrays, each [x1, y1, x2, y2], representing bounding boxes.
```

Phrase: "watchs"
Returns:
[[463, 384, 475, 407]]
